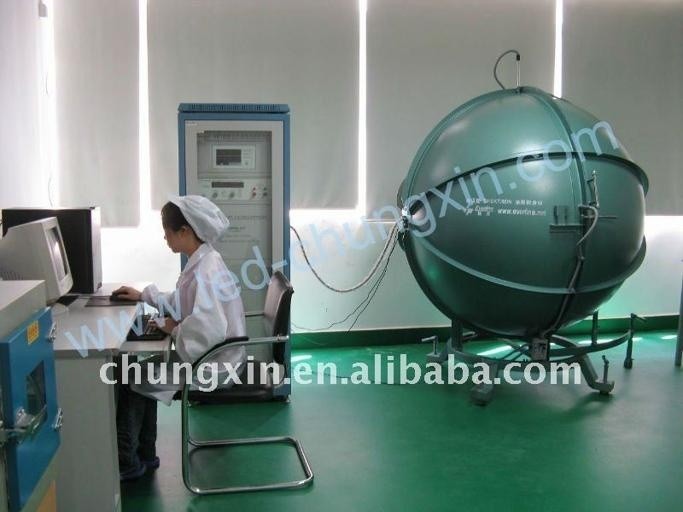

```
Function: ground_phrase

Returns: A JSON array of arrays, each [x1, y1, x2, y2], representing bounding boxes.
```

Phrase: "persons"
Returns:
[[112, 195, 246, 480]]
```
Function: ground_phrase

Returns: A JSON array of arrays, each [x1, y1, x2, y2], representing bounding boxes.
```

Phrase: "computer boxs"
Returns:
[[1, 205, 103, 294]]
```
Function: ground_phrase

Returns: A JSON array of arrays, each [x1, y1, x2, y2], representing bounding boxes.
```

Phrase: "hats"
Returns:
[[170, 195, 230, 243]]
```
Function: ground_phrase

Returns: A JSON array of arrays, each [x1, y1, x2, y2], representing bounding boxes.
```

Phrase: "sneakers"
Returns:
[[120, 456, 159, 480]]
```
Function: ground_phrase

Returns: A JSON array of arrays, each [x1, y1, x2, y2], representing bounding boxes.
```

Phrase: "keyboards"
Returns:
[[127, 313, 167, 341]]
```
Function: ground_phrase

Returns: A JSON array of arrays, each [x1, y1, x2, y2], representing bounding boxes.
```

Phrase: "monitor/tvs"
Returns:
[[0, 216, 74, 316]]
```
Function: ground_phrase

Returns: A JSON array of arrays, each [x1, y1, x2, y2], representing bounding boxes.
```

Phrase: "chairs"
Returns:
[[172, 270, 315, 496]]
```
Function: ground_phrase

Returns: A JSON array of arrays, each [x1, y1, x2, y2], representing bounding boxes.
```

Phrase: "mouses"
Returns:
[[109, 291, 129, 302]]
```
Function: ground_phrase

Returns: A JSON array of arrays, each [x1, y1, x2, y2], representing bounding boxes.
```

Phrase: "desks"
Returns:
[[51, 282, 172, 512]]
